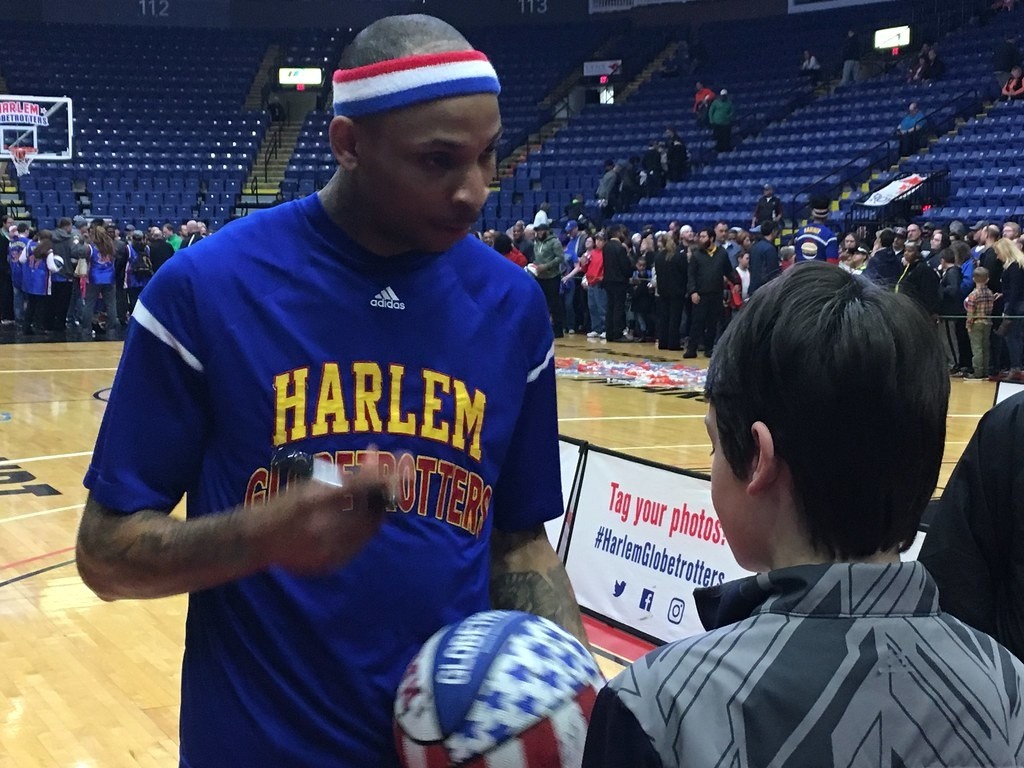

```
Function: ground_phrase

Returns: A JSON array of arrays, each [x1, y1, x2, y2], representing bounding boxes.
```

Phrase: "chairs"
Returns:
[[0, 0, 1024, 233]]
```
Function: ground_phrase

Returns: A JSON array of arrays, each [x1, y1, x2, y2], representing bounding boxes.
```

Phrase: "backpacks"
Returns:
[[128, 244, 152, 281]]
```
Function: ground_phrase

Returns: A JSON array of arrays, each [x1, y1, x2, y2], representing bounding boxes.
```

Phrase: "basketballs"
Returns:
[[390, 609, 604, 768]]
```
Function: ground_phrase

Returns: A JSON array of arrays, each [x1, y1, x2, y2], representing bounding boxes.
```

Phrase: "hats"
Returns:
[[749, 225, 762, 232], [594, 231, 606, 239], [924, 221, 935, 228], [679, 224, 692, 240], [533, 224, 550, 231], [893, 226, 908, 238], [565, 220, 578, 230], [969, 220, 989, 230]]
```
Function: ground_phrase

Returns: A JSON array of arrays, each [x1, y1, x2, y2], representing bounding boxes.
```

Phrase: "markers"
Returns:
[[271, 446, 390, 509]]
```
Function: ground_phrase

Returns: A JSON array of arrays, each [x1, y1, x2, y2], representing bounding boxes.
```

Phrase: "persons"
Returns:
[[564, 195, 586, 220], [74, 11, 604, 768], [840, 29, 860, 85], [999, 66, 1024, 102], [595, 126, 692, 219], [265, 97, 286, 121], [534, 203, 556, 227], [0, 215, 212, 336], [897, 103, 929, 159], [692, 81, 716, 129], [474, 200, 1024, 383], [751, 182, 783, 226], [583, 259, 1024, 768], [992, 0, 1014, 11], [919, 385, 1024, 666], [798, 49, 823, 88], [904, 42, 938, 83], [707, 89, 734, 152]]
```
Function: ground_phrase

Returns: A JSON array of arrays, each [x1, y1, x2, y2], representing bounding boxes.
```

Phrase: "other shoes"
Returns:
[[963, 374, 990, 380], [569, 329, 575, 334], [949, 363, 968, 376]]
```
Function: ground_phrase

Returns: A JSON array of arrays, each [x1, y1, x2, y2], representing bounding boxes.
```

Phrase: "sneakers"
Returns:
[[599, 331, 607, 338], [587, 331, 599, 338]]
